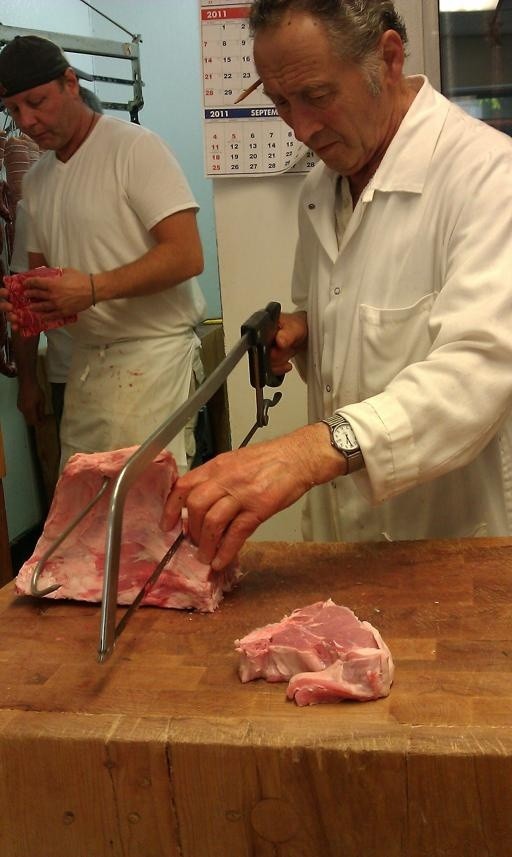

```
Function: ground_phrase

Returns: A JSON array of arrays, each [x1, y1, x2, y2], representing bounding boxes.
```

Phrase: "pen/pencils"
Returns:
[[234, 79, 263, 104]]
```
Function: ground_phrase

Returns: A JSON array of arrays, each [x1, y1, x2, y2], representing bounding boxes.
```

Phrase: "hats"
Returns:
[[0, 33, 95, 97]]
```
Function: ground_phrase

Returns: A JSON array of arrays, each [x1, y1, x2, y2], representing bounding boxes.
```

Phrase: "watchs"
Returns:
[[318, 414, 364, 476]]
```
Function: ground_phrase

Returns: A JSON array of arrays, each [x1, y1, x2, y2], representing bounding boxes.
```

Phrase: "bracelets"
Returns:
[[90, 271, 98, 307]]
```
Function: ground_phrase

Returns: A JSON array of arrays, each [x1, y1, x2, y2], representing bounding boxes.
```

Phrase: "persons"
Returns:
[[10, 325, 79, 451], [155, 1, 511, 575], [2, 31, 217, 479]]
